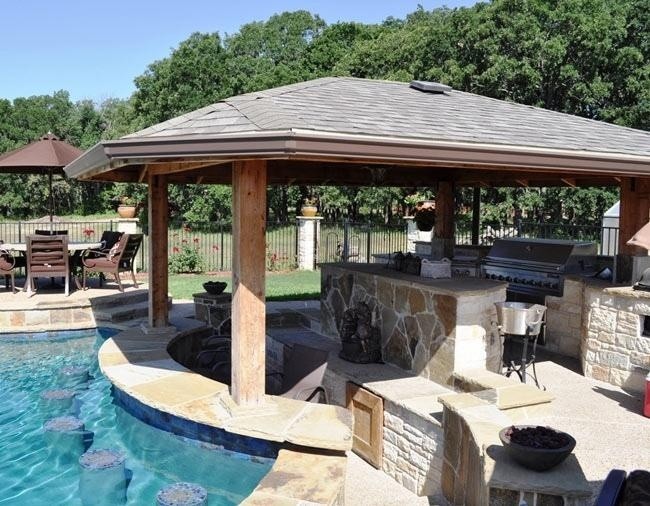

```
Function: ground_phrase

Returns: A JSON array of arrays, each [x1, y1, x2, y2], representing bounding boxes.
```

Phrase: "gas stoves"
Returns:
[[451, 255, 481, 278]]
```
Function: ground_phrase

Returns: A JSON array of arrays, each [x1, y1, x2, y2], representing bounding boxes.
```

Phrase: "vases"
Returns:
[[117, 205, 136, 219]]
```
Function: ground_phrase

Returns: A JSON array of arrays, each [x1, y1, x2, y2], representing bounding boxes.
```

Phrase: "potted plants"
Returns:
[[299, 195, 320, 218], [404, 193, 427, 217]]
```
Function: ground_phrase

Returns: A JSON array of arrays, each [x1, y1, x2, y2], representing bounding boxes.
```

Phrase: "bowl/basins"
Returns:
[[498, 425, 578, 473]]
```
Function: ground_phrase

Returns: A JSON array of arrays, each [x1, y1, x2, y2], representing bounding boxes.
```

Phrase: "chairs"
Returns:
[[0, 228, 143, 294]]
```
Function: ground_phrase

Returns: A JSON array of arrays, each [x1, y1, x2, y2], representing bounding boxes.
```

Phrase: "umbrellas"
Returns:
[[1, 131, 112, 235]]
